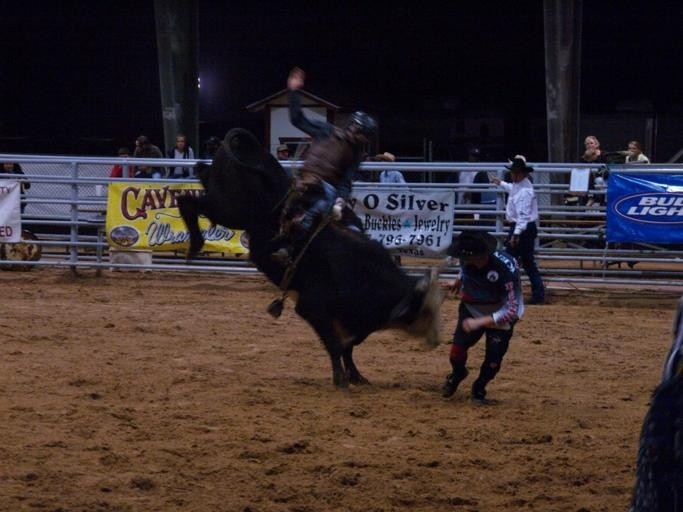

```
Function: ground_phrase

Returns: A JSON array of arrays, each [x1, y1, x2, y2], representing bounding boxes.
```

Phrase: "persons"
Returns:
[[491, 158, 545, 305], [110, 134, 197, 180], [414, 229, 525, 400], [0, 162, 31, 220], [376, 152, 405, 183], [274, 144, 289, 160], [456, 148, 527, 205], [580, 135, 653, 208], [268, 67, 381, 267]]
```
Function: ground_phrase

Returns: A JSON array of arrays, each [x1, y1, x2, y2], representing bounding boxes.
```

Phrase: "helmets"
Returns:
[[345, 110, 378, 144]]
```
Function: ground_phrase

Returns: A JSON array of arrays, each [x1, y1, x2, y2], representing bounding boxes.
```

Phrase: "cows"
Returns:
[[173, 124, 441, 390]]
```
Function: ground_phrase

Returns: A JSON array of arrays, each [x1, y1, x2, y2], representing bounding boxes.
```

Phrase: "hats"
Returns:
[[376, 152, 395, 162], [504, 155, 534, 173], [447, 229, 497, 259]]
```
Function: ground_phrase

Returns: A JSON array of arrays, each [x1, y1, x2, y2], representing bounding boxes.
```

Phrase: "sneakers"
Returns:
[[473, 382, 486, 400], [442, 369, 469, 398]]
